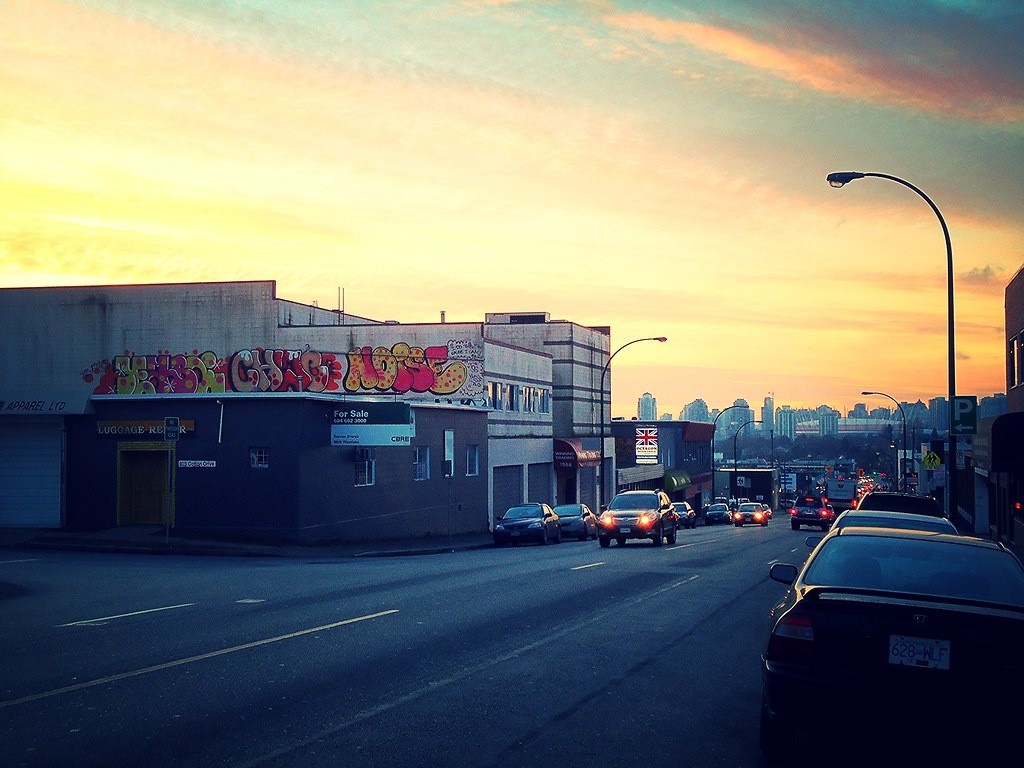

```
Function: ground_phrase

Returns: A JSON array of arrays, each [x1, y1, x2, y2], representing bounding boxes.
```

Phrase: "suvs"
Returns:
[[596, 489, 681, 547], [789, 495, 838, 532]]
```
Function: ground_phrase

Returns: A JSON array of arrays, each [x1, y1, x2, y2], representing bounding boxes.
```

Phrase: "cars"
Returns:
[[762, 503, 773, 519], [760, 526, 1024, 768], [671, 500, 697, 529], [704, 502, 733, 525], [804, 475, 959, 550], [493, 502, 562, 547], [734, 502, 769, 527], [551, 503, 600, 542], [713, 495, 750, 510]]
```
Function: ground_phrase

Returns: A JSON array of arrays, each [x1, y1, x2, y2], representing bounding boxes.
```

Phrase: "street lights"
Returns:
[[600, 336, 668, 511], [711, 405, 749, 503], [861, 391, 907, 493], [732, 421, 765, 500], [784, 445, 800, 513], [828, 171, 956, 533]]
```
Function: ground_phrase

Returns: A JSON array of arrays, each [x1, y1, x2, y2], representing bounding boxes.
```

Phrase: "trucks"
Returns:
[[828, 479, 861, 514]]
